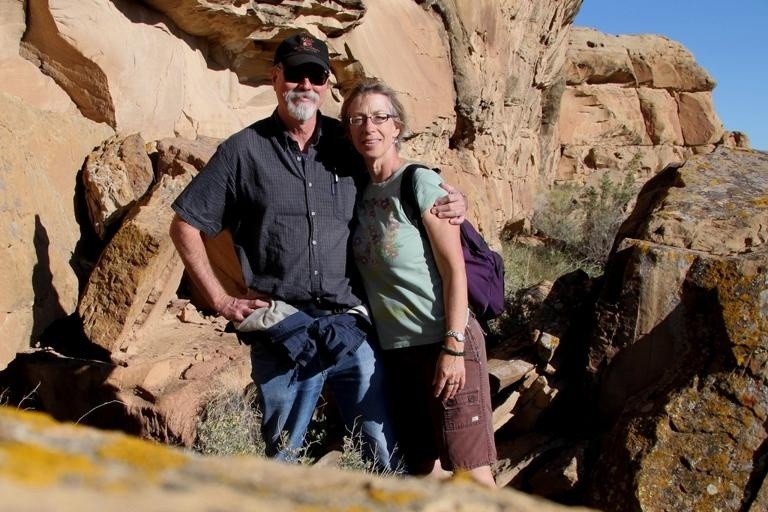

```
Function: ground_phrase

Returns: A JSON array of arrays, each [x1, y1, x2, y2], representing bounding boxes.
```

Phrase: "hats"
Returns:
[[273, 35, 329, 71]]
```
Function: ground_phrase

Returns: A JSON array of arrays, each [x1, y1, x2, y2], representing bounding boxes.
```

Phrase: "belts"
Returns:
[[306, 306, 349, 318]]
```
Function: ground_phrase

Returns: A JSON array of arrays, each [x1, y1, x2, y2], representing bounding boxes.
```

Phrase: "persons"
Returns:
[[339, 79, 497, 487], [170, 32, 467, 473]]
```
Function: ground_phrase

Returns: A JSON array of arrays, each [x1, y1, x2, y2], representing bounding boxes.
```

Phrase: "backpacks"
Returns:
[[460, 219, 505, 320]]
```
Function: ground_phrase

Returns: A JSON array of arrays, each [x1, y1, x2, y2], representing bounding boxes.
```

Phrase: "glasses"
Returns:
[[346, 113, 399, 126], [277, 63, 330, 86]]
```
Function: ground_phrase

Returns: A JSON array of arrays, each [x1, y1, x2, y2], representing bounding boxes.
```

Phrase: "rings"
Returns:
[[447, 382, 455, 385], [456, 382, 460, 385]]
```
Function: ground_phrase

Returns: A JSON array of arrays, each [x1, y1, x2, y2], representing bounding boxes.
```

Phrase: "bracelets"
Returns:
[[441, 345, 465, 356]]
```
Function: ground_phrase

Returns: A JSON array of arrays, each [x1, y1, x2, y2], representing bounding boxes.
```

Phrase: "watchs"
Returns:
[[444, 330, 465, 342]]
